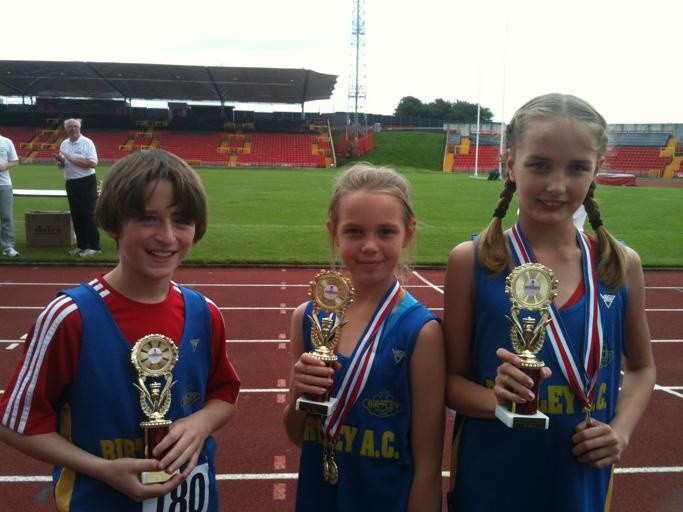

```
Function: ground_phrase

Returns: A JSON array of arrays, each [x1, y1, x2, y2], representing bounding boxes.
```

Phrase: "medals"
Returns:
[[327, 454, 339, 487], [320, 451, 330, 484]]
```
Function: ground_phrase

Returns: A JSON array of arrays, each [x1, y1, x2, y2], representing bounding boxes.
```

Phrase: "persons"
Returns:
[[0, 146, 242, 512], [281, 159, 449, 511], [49, 118, 103, 257], [440, 92, 655, 510], [0, 134, 20, 258]]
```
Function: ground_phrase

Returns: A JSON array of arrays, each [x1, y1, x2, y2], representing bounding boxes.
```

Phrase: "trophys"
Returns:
[[293, 265, 354, 419], [491, 262, 559, 432], [130, 331, 181, 486]]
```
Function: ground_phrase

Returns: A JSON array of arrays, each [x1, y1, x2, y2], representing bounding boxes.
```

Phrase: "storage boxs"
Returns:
[[23, 210, 73, 248]]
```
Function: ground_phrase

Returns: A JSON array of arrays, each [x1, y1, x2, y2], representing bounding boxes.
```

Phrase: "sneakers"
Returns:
[[68, 247, 102, 257], [2, 247, 20, 258]]
